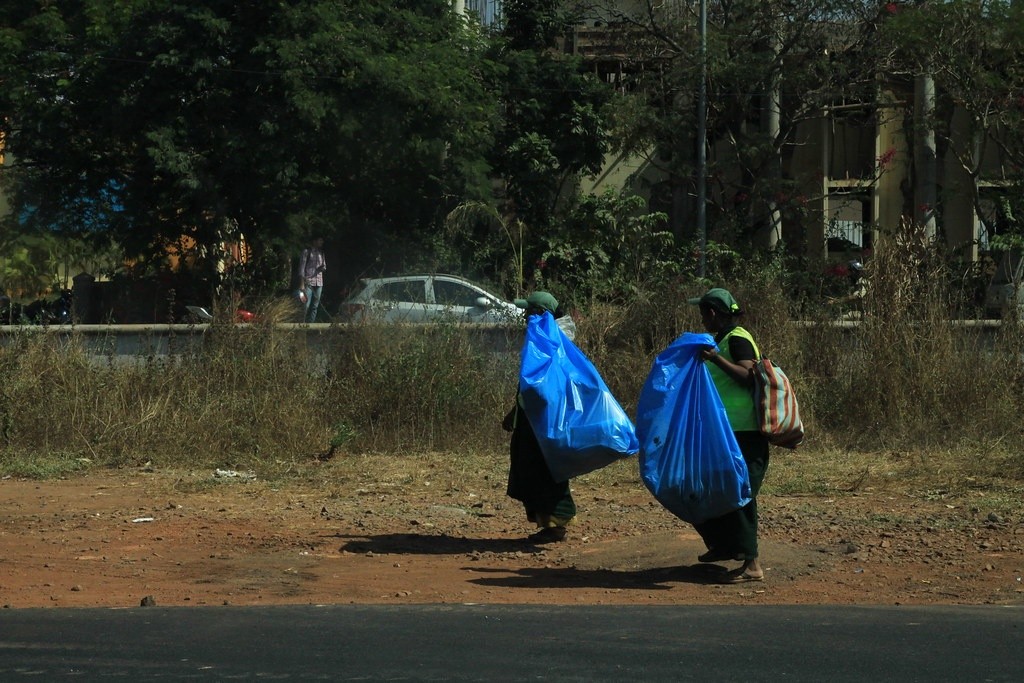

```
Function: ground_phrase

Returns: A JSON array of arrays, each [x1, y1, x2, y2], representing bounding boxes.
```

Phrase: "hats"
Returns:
[[689, 287, 739, 314], [513, 290, 559, 315]]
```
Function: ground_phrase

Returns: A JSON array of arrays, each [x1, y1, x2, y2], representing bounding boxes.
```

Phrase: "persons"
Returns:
[[299, 237, 326, 323], [695, 288, 770, 584], [506, 292, 576, 544]]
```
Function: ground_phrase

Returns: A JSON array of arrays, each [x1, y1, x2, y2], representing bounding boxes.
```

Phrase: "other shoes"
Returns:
[[725, 567, 764, 583], [527, 525, 566, 542], [697, 548, 730, 562]]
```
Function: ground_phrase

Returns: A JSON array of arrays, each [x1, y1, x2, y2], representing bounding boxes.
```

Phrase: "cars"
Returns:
[[340, 272, 529, 325]]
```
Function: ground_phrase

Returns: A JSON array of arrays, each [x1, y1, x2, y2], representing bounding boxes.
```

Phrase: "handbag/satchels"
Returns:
[[748, 354, 804, 449]]
